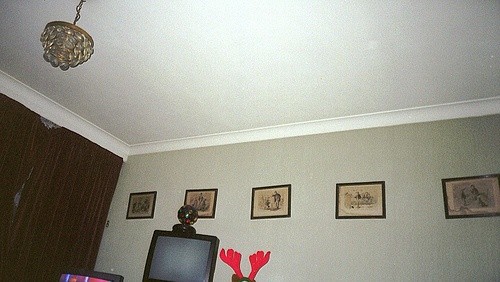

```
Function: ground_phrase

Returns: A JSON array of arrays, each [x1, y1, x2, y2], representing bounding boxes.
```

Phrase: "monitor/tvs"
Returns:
[[142, 229, 220, 282], [54, 266, 124, 282]]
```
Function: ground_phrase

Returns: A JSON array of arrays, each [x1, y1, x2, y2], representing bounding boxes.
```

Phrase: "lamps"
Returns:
[[38, 0, 95, 71]]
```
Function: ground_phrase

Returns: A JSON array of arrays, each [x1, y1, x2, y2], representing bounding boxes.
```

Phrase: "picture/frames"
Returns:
[[251, 184, 291, 220], [335, 181, 387, 220], [124, 190, 158, 220], [441, 173, 500, 220], [184, 187, 218, 219]]
